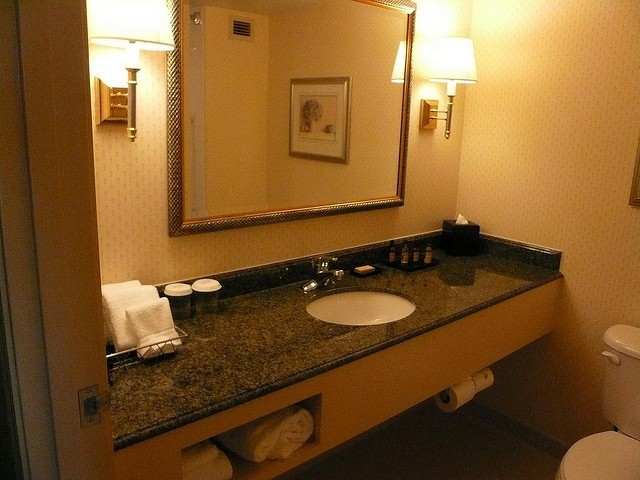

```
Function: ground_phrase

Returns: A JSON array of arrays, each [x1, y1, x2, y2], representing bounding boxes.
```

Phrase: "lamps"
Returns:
[[428, 34, 481, 140], [88, 0, 175, 143]]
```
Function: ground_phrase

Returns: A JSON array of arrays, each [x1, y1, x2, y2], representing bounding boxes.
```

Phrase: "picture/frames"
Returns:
[[286, 75, 351, 166]]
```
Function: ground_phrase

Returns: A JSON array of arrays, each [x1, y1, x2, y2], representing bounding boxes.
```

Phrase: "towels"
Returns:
[[182, 439, 233, 479], [214, 405, 315, 463], [103, 280, 140, 345], [103, 285, 159, 352], [126, 297, 181, 357]]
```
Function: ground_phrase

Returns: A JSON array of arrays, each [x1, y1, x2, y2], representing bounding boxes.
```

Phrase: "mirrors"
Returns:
[[167, 2, 419, 237]]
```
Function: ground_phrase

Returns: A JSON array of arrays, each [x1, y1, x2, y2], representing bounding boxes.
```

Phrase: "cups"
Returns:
[[191, 279, 222, 313], [165, 282, 192, 321]]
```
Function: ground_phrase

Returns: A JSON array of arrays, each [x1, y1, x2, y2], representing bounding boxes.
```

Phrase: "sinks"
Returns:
[[305, 286, 417, 327]]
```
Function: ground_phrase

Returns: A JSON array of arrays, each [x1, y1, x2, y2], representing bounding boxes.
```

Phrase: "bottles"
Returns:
[[413, 242, 421, 266], [423, 243, 433, 265], [388, 239, 397, 265], [401, 239, 410, 267]]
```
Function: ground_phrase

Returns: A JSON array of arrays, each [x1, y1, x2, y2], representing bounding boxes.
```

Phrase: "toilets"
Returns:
[[553, 323, 640, 479]]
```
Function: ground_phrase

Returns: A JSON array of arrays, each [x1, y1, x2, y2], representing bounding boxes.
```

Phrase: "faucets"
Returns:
[[301, 254, 345, 294]]
